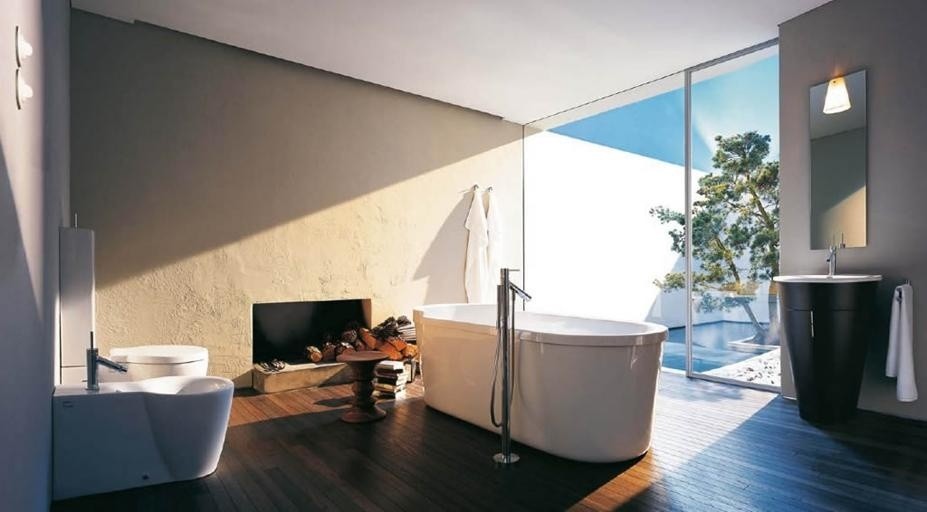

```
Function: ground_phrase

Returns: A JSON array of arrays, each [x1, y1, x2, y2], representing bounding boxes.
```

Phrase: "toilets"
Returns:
[[59, 222, 209, 382]]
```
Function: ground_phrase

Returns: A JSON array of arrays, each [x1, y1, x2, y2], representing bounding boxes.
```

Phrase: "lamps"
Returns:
[[823, 77, 851, 114]]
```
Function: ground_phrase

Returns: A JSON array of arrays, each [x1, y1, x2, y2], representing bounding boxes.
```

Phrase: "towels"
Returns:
[[464, 190, 487, 303], [885, 283, 918, 402], [486, 190, 504, 275]]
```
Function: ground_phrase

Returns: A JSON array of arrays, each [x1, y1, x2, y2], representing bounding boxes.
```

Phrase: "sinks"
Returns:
[[128, 375, 234, 406], [773, 273, 882, 286]]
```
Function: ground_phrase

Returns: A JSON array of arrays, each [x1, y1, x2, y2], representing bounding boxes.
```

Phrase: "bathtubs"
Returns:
[[414, 303, 669, 466]]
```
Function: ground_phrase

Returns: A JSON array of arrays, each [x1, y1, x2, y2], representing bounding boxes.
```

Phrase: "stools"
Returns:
[[335, 351, 390, 423]]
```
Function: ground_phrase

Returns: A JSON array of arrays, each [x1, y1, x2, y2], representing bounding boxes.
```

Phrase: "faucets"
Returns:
[[823, 245, 837, 276], [496, 268, 532, 305], [83, 330, 128, 393]]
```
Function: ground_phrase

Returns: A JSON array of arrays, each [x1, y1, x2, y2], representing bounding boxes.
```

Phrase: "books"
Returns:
[[372, 357, 416, 400], [397, 324, 417, 342]]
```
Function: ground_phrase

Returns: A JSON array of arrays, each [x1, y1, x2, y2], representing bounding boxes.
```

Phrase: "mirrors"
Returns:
[[809, 69, 867, 250]]
